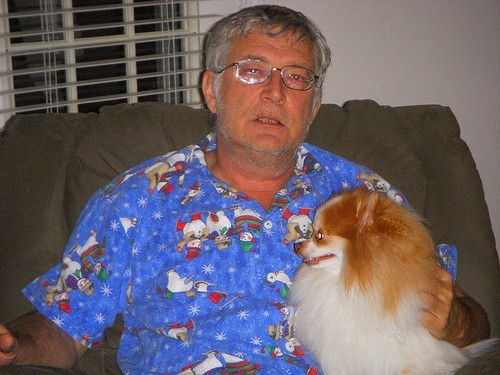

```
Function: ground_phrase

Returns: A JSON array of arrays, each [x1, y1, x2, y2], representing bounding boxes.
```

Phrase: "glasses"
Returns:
[[215, 57, 320, 92]]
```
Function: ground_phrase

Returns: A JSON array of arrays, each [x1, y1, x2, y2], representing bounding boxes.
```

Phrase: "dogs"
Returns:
[[286, 182, 500, 375]]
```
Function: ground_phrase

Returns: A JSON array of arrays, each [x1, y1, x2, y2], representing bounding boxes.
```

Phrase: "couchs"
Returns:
[[0, 99, 500, 375]]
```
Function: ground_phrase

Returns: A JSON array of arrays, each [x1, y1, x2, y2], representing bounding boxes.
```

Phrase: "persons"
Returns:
[[1, 7, 492, 373]]
